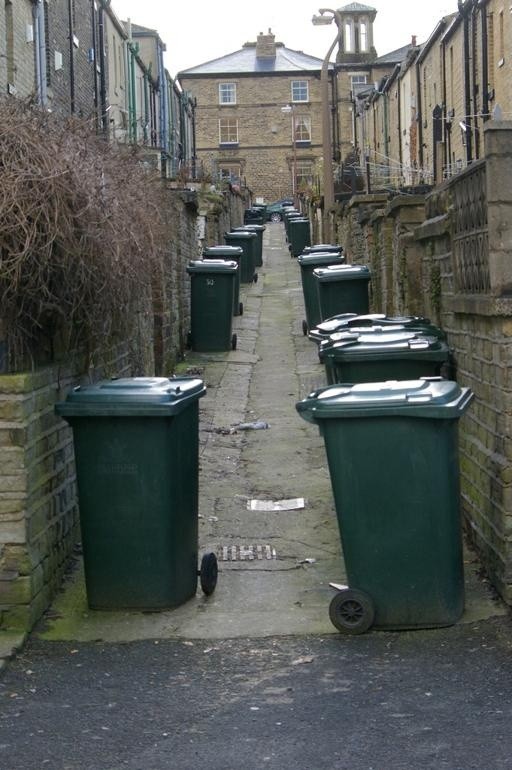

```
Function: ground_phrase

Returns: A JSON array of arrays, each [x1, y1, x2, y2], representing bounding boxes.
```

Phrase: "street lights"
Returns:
[[312, 8, 343, 217]]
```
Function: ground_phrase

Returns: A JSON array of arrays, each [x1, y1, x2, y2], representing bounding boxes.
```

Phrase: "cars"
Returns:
[[265, 200, 293, 224]]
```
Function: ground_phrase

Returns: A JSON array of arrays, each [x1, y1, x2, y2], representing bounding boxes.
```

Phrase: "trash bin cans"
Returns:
[[53, 371, 219, 612], [305, 313, 456, 387], [189, 202, 379, 351], [293, 377, 476, 635]]
